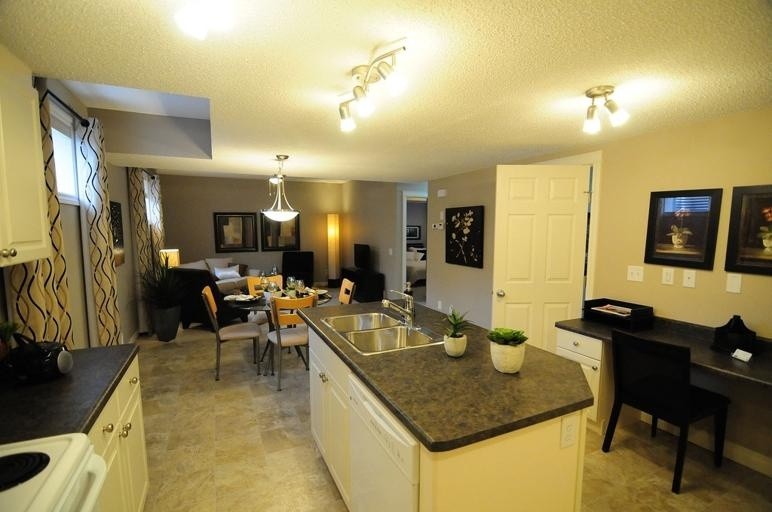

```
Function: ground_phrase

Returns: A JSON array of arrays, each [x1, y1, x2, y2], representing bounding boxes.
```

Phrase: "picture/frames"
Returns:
[[214, 211, 301, 253], [405, 225, 421, 240], [643, 185, 771, 278]]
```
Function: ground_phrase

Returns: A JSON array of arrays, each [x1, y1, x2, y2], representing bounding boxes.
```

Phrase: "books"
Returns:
[[590, 303, 633, 318]]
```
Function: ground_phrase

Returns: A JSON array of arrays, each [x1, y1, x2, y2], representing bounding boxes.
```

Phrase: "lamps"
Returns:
[[326, 213, 340, 288], [159, 248, 179, 270], [335, 38, 406, 136], [581, 81, 630, 136], [261, 151, 300, 223]]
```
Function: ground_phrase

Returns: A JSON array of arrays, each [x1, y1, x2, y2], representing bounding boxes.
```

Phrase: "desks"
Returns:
[[548, 297, 771, 494]]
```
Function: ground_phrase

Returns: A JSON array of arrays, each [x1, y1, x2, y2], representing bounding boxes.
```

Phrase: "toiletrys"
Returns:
[[404, 282, 413, 297]]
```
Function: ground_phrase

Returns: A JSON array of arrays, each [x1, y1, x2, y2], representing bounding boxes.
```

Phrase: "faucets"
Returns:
[[381, 290, 416, 321]]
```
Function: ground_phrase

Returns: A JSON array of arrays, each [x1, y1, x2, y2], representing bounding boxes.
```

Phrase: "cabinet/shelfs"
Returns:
[[310, 326, 422, 512], [557, 328, 641, 424], [339, 266, 384, 303], [75, 353, 148, 510], [0, 83, 53, 267]]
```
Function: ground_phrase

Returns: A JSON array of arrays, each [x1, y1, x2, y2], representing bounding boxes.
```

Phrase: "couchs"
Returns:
[[176, 257, 260, 295]]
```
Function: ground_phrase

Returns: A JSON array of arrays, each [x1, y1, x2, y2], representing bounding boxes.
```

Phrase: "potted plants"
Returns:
[[442, 305, 528, 374], [134, 266, 189, 341]]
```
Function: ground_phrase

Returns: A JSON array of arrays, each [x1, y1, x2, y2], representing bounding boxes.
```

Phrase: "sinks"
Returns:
[[345, 327, 433, 352], [320, 312, 399, 331]]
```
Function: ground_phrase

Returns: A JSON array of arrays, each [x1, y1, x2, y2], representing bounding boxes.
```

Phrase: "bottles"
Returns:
[[261, 263, 278, 277]]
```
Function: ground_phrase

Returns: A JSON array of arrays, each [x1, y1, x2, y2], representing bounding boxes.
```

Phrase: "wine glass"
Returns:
[[286, 276, 305, 298], [261, 278, 278, 299]]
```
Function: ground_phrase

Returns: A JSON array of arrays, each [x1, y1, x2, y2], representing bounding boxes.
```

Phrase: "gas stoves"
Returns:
[[1, 432, 95, 511]]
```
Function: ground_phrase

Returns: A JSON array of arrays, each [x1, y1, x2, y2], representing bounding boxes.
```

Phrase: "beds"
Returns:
[[405, 242, 426, 287]]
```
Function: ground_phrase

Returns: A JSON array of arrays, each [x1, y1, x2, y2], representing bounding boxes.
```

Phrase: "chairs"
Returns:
[[201, 271, 358, 392], [600, 328, 730, 492]]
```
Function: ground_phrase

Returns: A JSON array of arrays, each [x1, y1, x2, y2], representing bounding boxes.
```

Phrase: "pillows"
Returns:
[[182, 257, 247, 279], [406, 246, 427, 263]]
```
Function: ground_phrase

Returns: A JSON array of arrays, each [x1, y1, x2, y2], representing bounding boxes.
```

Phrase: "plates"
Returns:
[[316, 289, 328, 295]]
